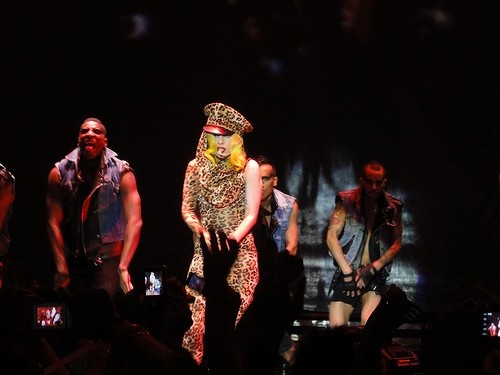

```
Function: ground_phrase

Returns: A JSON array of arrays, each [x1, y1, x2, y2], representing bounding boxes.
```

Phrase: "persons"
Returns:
[[0, 162, 15, 256], [0, 282, 200, 375], [246, 154, 299, 255], [47, 118, 143, 294], [198, 228, 500, 375], [326, 160, 402, 328], [182, 102, 262, 366]]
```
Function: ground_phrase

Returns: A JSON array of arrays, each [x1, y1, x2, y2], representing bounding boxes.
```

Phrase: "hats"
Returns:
[[203, 103, 254, 136]]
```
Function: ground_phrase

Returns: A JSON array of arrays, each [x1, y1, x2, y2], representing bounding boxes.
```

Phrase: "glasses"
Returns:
[[366, 178, 384, 186]]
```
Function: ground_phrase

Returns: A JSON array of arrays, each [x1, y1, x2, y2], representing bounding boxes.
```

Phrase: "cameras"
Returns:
[[32, 303, 74, 330], [480, 310, 500, 339]]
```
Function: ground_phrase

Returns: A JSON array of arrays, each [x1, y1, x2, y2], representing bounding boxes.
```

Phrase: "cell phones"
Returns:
[[187, 273, 206, 296], [144, 270, 164, 298]]
